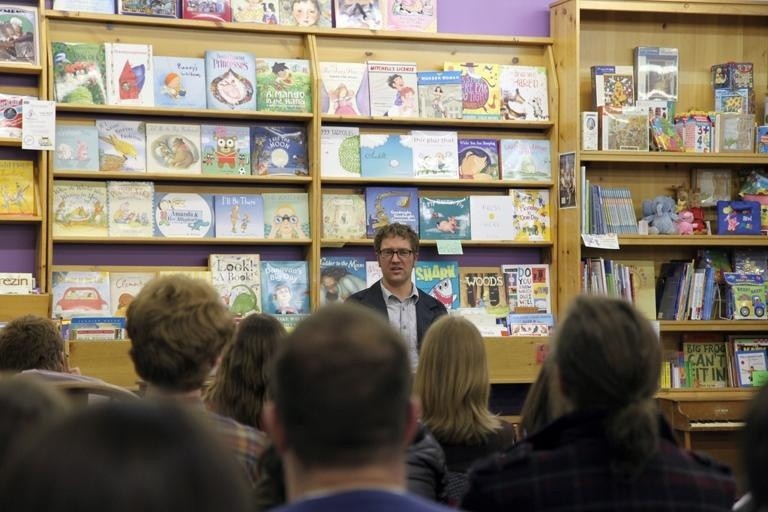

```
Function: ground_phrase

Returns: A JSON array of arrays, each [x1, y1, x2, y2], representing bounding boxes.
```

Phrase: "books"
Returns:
[[0, 0, 555, 337], [579, 45, 768, 390]]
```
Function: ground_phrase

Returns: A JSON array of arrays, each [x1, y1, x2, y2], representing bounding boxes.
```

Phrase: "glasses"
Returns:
[[379, 247, 415, 258]]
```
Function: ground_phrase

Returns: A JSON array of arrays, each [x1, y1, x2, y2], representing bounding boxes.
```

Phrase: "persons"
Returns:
[[122, 271, 283, 510], [345, 223, 449, 376], [1, 373, 259, 511], [456, 289, 736, 512], [0, 314, 81, 375], [411, 312, 520, 510], [200, 310, 294, 432], [0, 377, 70, 466], [257, 298, 459, 512], [516, 348, 564, 442], [725, 370, 768, 512]]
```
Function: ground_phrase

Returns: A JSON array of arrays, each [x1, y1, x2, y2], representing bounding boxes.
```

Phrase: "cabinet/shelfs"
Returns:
[[43, 10, 314, 392], [0, 0, 50, 328], [312, 28, 558, 384], [550, 0, 768, 392]]
